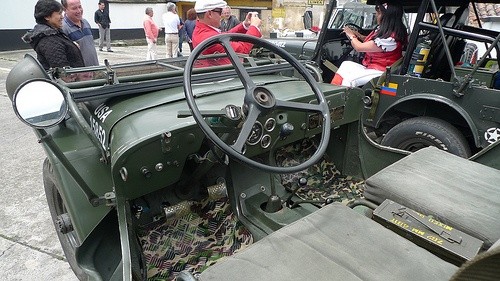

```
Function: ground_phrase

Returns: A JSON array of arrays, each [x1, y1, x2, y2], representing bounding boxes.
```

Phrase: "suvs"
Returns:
[[249, 0, 500, 171], [4, 32, 500, 281]]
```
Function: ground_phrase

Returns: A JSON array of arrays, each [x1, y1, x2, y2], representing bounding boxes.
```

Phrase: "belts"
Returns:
[[165, 33, 178, 34]]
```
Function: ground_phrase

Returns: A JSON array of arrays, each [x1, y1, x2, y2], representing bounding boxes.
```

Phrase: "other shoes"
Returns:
[[108, 50, 113, 52]]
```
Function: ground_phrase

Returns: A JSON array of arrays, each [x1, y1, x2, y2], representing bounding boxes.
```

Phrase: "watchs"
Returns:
[[348, 35, 357, 41]]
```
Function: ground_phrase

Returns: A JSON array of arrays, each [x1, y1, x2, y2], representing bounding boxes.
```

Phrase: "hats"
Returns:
[[194, 0, 227, 13]]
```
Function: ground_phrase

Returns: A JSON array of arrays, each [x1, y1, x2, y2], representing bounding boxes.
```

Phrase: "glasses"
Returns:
[[211, 8, 222, 16]]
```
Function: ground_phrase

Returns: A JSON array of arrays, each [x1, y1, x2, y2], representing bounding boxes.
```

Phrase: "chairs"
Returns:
[[198, 145, 500, 281]]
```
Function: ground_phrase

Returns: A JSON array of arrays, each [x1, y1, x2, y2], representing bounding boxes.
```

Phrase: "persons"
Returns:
[[94, 1, 114, 52], [183, 8, 198, 52], [174, 8, 185, 56], [143, 7, 162, 60], [331, 0, 408, 88], [61, 0, 100, 67], [192, 0, 262, 68], [220, 6, 240, 32], [22, 0, 85, 78], [161, 2, 180, 59]]
